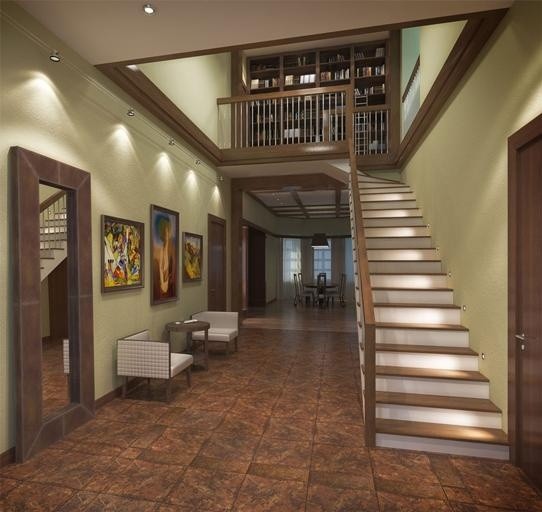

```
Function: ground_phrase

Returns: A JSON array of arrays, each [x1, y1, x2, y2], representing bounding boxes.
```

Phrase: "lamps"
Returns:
[[311, 233, 330, 250]]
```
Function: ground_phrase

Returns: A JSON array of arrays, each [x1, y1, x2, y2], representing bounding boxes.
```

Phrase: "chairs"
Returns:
[[293, 273, 346, 308], [190, 311, 239, 357], [117, 329, 193, 404]]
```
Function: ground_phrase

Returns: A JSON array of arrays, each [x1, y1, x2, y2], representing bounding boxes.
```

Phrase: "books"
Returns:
[[249, 48, 386, 155]]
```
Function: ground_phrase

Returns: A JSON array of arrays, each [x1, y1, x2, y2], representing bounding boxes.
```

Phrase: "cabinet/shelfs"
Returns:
[[246, 38, 388, 154]]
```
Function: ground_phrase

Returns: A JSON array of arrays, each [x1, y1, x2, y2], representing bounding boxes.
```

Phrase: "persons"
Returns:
[[152, 216, 177, 299]]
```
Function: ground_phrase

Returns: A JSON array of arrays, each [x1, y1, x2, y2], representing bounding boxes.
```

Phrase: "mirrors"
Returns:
[[8, 146, 96, 464]]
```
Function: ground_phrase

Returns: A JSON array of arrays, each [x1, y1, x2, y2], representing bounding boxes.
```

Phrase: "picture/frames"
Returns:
[[182, 232, 204, 283], [150, 204, 180, 305], [101, 215, 145, 293]]
